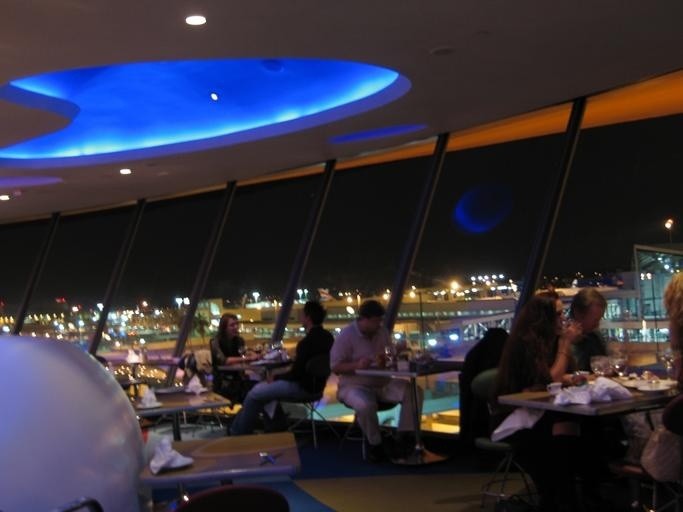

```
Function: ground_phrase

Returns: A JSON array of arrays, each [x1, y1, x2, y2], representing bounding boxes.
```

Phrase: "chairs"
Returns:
[[471, 367, 538, 509], [288, 368, 340, 450], [344, 402, 395, 441]]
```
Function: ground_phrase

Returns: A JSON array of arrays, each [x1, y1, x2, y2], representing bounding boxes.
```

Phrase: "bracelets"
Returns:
[[557, 349, 570, 359]]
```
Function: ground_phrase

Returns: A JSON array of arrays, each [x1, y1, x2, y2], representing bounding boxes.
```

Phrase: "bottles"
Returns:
[[384, 344, 396, 367]]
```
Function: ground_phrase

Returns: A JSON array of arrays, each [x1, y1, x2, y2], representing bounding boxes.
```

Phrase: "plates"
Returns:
[[612, 376, 678, 392], [136, 401, 162, 409]]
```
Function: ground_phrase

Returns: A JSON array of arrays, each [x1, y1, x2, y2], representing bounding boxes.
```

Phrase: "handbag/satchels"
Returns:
[[640, 428, 683, 482]]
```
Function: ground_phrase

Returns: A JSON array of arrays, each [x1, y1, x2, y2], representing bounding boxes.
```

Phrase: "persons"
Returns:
[[329, 299, 429, 461], [229, 299, 334, 436], [488, 291, 583, 511], [210, 312, 299, 433], [639, 271, 683, 484], [567, 288, 627, 511]]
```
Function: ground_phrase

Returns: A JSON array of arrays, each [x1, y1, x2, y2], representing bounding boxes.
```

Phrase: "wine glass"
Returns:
[[589, 350, 629, 378], [238, 343, 280, 361]]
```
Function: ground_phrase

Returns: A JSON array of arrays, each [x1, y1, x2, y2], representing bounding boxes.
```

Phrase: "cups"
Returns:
[[546, 382, 562, 396]]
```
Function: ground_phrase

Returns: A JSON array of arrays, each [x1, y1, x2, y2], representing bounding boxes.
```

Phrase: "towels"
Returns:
[[491, 408, 545, 442], [184, 373, 207, 395], [149, 435, 194, 475], [552, 373, 634, 406], [142, 387, 157, 405]]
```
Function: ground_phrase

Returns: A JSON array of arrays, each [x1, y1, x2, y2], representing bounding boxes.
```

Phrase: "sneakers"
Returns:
[[366, 435, 418, 471]]
